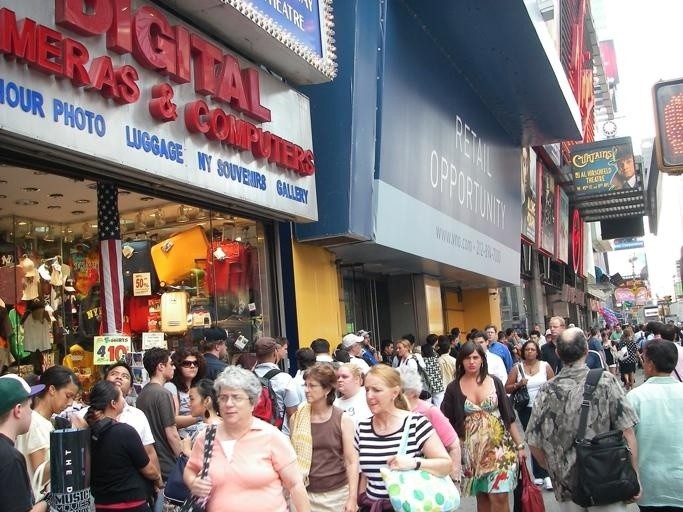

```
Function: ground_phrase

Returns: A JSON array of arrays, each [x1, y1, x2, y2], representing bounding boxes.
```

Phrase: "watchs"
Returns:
[[413, 455, 422, 470], [176, 452, 184, 461]]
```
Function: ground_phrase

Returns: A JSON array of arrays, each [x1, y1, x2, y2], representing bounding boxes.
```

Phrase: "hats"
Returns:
[[341, 333, 364, 349], [356, 329, 371, 337], [204, 327, 229, 341], [22, 258, 35, 277], [38, 264, 52, 281], [0, 373, 45, 420], [254, 337, 280, 356]]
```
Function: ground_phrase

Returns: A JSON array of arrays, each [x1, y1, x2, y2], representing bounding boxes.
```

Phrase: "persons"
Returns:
[[544, 328, 552, 342], [71, 359, 161, 474], [534, 325, 547, 350], [281, 347, 316, 437], [524, 326, 643, 512], [394, 365, 462, 497], [588, 327, 606, 362], [295, 338, 334, 379], [514, 331, 523, 345], [609, 323, 623, 340], [78, 380, 164, 512], [540, 315, 565, 376], [627, 339, 683, 512], [451, 327, 462, 351], [668, 320, 679, 333], [504, 334, 519, 364], [13, 365, 81, 503], [497, 333, 506, 345], [609, 146, 636, 190], [201, 326, 229, 382], [438, 335, 449, 347], [353, 363, 453, 512], [252, 336, 300, 431], [426, 334, 438, 352], [505, 340, 555, 432], [289, 363, 360, 512], [529, 330, 542, 351], [484, 323, 514, 375], [163, 347, 209, 440], [183, 365, 312, 512], [466, 333, 473, 342], [332, 349, 353, 364], [659, 323, 683, 383], [392, 333, 416, 368], [440, 340, 527, 512], [679, 321, 683, 346], [275, 336, 290, 364], [381, 340, 394, 367], [616, 327, 640, 390], [448, 335, 458, 359], [438, 340, 456, 393], [470, 330, 511, 398], [601, 330, 617, 377], [636, 331, 651, 356], [0, 373, 48, 512], [179, 378, 222, 459], [521, 146, 537, 235], [644, 322, 664, 359], [623, 322, 630, 328], [356, 330, 379, 367], [332, 363, 374, 472], [505, 328, 522, 357], [421, 344, 444, 409], [634, 324, 647, 343], [646, 321, 655, 340], [336, 333, 370, 374], [470, 328, 478, 334], [396, 338, 419, 372]]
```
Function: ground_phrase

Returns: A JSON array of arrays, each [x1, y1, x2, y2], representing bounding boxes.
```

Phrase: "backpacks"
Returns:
[[406, 354, 434, 400], [252, 368, 284, 428]]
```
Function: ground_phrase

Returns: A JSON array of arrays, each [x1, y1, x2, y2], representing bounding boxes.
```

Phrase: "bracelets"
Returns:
[[514, 383, 518, 390], [515, 443, 525, 451], [196, 415, 203, 422], [43, 498, 52, 510]]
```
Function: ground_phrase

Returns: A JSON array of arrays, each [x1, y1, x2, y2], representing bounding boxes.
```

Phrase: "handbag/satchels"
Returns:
[[509, 363, 530, 412], [520, 457, 545, 511], [380, 413, 461, 512], [569, 369, 639, 507], [161, 426, 216, 512], [614, 340, 629, 362], [34, 461, 53, 507]]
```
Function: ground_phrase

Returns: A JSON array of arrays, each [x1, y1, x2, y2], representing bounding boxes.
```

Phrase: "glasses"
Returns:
[[178, 360, 199, 369], [218, 395, 255, 401], [301, 383, 324, 388], [161, 361, 175, 367]]
